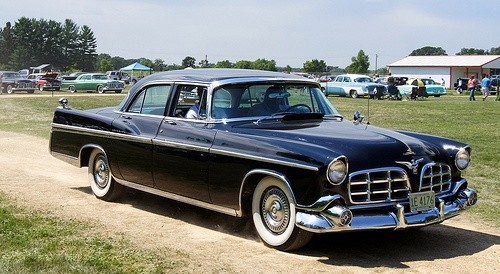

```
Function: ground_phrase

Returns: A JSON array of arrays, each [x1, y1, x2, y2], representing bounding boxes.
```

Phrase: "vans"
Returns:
[[489, 75, 500, 95], [457, 78, 481, 91], [290, 71, 316, 81]]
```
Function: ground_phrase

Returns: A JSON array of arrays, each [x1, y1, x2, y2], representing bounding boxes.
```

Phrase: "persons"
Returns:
[[371, 72, 491, 102], [184, 86, 291, 120]]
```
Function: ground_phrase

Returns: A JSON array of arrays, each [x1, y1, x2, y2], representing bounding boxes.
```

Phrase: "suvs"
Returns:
[[105, 71, 137, 84]]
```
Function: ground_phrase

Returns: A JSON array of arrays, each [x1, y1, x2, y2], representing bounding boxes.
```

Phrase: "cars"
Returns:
[[317, 76, 336, 82], [387, 77, 447, 97], [319, 74, 387, 99], [62, 72, 100, 80], [0, 71, 40, 94], [48, 68, 478, 250], [61, 74, 126, 94], [26, 72, 62, 91]]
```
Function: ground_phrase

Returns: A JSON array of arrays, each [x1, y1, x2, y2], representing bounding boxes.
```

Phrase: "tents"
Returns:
[[119, 62, 154, 82]]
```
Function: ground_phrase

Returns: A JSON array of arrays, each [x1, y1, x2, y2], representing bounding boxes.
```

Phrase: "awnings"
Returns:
[[31, 63, 51, 74]]
